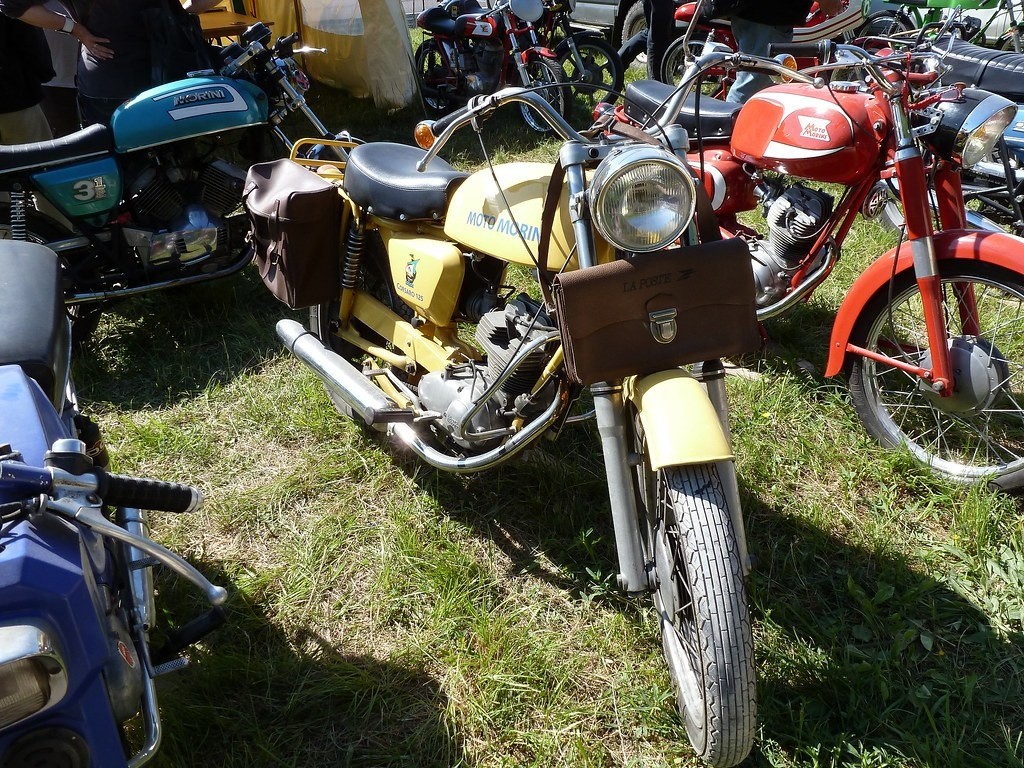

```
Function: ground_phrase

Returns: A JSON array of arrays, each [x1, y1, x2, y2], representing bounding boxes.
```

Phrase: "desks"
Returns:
[[198, 11, 273, 47]]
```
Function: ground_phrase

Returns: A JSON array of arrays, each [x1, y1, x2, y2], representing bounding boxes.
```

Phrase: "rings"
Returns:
[[94, 44, 98, 48]]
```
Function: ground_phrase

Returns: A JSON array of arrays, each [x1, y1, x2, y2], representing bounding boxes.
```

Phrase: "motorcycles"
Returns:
[[0, 239, 227, 768], [659, 0, 919, 103], [545, 1, 624, 118], [224, 36, 823, 767], [855, 21, 1024, 242], [589, 37, 1024, 494], [0, 15, 369, 316], [412, 0, 569, 137]]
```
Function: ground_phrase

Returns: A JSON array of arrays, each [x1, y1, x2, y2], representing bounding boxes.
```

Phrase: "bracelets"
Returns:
[[55, 16, 75, 36]]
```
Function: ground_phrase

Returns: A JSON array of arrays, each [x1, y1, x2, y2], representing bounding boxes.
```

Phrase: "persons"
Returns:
[[0, 0, 223, 147], [724, 0, 815, 103], [599, 0, 677, 92]]
